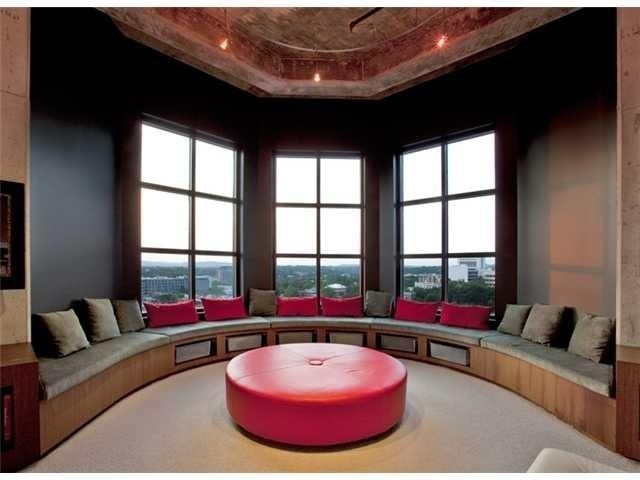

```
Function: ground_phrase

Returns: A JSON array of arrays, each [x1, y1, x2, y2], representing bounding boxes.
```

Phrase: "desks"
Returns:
[[616, 346, 640, 465], [2, 342, 38, 473]]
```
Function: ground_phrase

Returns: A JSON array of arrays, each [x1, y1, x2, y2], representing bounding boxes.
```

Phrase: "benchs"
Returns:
[[38, 331, 172, 460], [370, 312, 505, 386], [265, 316, 373, 352], [139, 317, 271, 375], [480, 332, 616, 454]]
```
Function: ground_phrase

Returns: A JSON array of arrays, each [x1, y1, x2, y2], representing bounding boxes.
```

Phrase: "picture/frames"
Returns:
[[1, 180, 25, 290]]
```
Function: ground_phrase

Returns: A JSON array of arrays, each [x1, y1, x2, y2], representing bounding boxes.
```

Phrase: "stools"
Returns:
[[522, 446, 624, 472], [226, 343, 407, 446]]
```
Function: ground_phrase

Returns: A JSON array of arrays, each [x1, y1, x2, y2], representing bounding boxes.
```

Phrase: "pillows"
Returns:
[[439, 301, 494, 330], [567, 314, 615, 363], [497, 303, 531, 337], [319, 296, 364, 316], [202, 297, 248, 320], [521, 302, 566, 346], [276, 295, 319, 317], [79, 296, 121, 345], [393, 297, 440, 323], [248, 288, 277, 317], [364, 290, 393, 318], [32, 308, 90, 359], [144, 300, 200, 326], [111, 299, 145, 333]]
